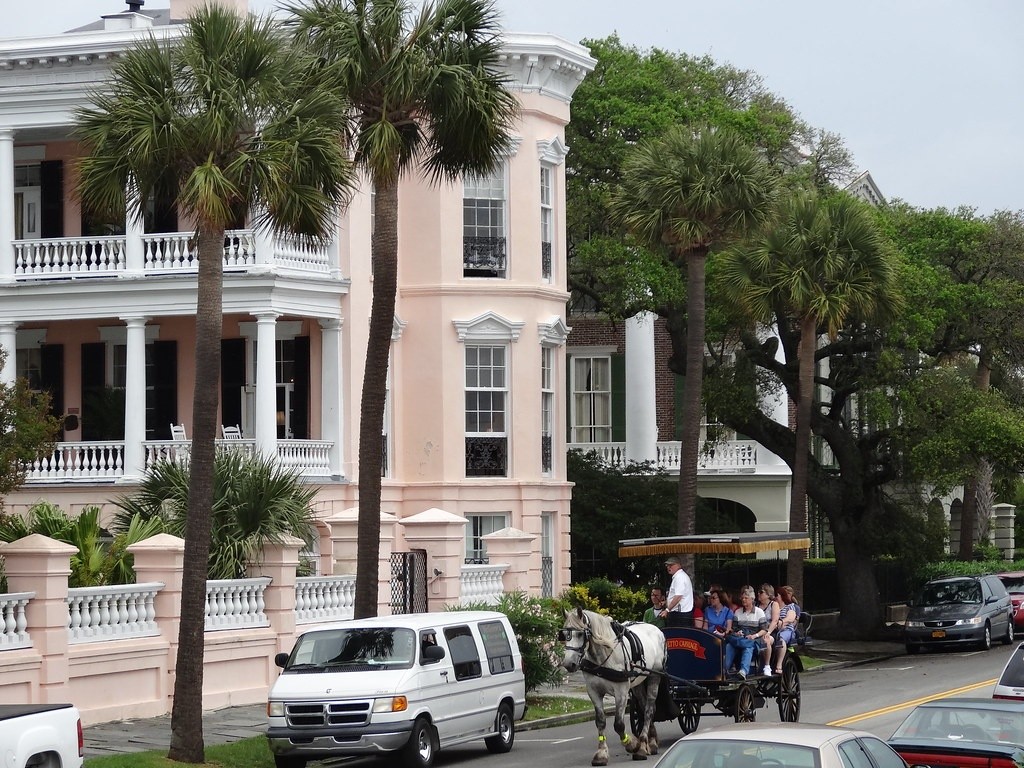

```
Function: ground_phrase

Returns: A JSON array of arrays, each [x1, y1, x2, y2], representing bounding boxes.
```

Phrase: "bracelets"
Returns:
[[665, 608, 670, 613]]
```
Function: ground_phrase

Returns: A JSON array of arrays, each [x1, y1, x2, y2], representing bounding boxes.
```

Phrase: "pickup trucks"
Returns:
[[0, 700, 85, 768]]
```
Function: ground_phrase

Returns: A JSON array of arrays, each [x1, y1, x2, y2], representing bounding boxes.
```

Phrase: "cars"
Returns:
[[655, 722, 931, 768], [995, 572, 1024, 633], [886, 696, 1024, 768]]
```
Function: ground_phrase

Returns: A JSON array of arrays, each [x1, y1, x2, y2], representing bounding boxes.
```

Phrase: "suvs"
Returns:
[[903, 572, 1014, 655], [992, 641, 1024, 701]]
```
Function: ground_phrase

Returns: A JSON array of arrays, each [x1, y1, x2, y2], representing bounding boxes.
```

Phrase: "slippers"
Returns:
[[775, 668, 783, 674]]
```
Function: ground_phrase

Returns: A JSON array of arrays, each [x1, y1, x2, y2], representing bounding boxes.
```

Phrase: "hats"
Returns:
[[665, 557, 681, 564]]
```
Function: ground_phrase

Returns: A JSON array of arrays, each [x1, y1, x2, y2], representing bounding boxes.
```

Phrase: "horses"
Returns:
[[559, 606, 669, 767]]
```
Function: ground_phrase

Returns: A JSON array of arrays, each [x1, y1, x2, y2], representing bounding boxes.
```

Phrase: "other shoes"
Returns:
[[737, 670, 747, 680]]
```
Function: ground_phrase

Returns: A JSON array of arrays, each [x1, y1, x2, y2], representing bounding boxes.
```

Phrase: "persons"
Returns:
[[658, 556, 694, 628], [642, 585, 667, 633], [692, 583, 801, 681]]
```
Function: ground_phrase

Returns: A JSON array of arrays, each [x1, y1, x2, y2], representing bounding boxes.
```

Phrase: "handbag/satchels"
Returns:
[[714, 625, 727, 637], [726, 628, 755, 647]]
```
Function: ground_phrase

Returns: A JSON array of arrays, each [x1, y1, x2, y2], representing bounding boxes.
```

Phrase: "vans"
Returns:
[[264, 610, 526, 766]]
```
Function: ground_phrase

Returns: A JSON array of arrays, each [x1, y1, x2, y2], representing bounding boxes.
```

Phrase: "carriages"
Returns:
[[559, 530, 812, 765]]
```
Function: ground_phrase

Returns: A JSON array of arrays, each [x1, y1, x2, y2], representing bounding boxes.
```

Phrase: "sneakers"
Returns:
[[764, 664, 772, 675]]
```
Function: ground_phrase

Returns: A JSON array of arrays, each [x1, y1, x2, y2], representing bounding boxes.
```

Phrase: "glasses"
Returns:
[[710, 596, 719, 598], [759, 591, 766, 594], [666, 564, 677, 566], [710, 590, 717, 593]]
